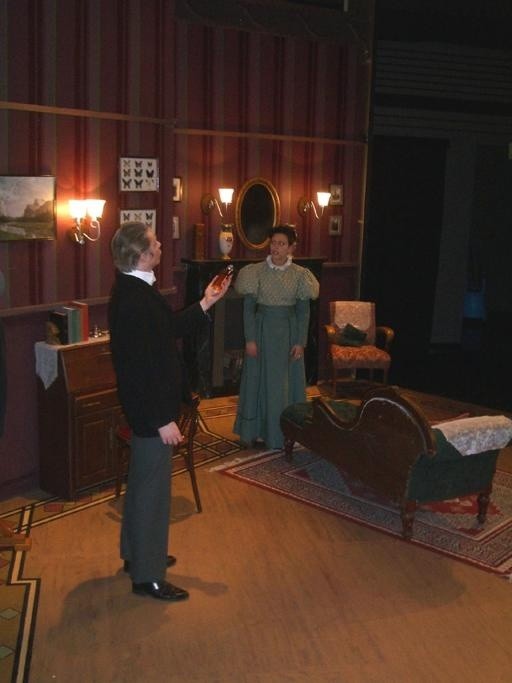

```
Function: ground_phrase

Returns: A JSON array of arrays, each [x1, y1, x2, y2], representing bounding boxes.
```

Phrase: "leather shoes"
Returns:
[[123, 556, 176, 572], [139, 580, 188, 600]]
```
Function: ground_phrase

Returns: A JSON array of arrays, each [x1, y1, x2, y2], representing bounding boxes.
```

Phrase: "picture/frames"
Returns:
[[329, 183, 345, 207], [172, 216, 180, 240], [118, 208, 158, 235], [117, 155, 160, 192], [0, 175, 57, 242], [173, 176, 182, 201], [327, 214, 344, 237]]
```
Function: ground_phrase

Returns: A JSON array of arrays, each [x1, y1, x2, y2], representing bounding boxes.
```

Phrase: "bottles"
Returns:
[[210, 264, 233, 291]]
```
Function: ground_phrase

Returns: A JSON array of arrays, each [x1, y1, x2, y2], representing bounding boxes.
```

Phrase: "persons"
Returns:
[[108, 222, 233, 602], [233, 225, 320, 452]]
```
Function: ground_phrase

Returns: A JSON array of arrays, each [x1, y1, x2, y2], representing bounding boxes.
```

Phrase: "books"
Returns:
[[51, 300, 88, 345]]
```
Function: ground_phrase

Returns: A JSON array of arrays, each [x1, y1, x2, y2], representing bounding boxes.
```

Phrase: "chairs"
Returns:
[[319, 299, 395, 396], [115, 392, 203, 512]]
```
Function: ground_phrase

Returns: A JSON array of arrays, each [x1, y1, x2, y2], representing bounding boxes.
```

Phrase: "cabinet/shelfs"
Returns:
[[34, 331, 129, 502], [181, 257, 328, 399]]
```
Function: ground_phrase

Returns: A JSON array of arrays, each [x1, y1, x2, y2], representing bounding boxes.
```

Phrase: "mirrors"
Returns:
[[235, 177, 280, 251]]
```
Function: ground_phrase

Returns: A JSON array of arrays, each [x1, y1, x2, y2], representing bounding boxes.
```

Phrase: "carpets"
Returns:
[[214, 444, 512, 579]]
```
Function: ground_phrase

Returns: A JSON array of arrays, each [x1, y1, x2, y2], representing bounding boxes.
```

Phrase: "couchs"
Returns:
[[280, 385, 512, 541]]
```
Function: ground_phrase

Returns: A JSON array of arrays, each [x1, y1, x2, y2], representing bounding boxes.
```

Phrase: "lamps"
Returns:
[[297, 192, 331, 219], [201, 188, 234, 219], [66, 200, 106, 248]]
